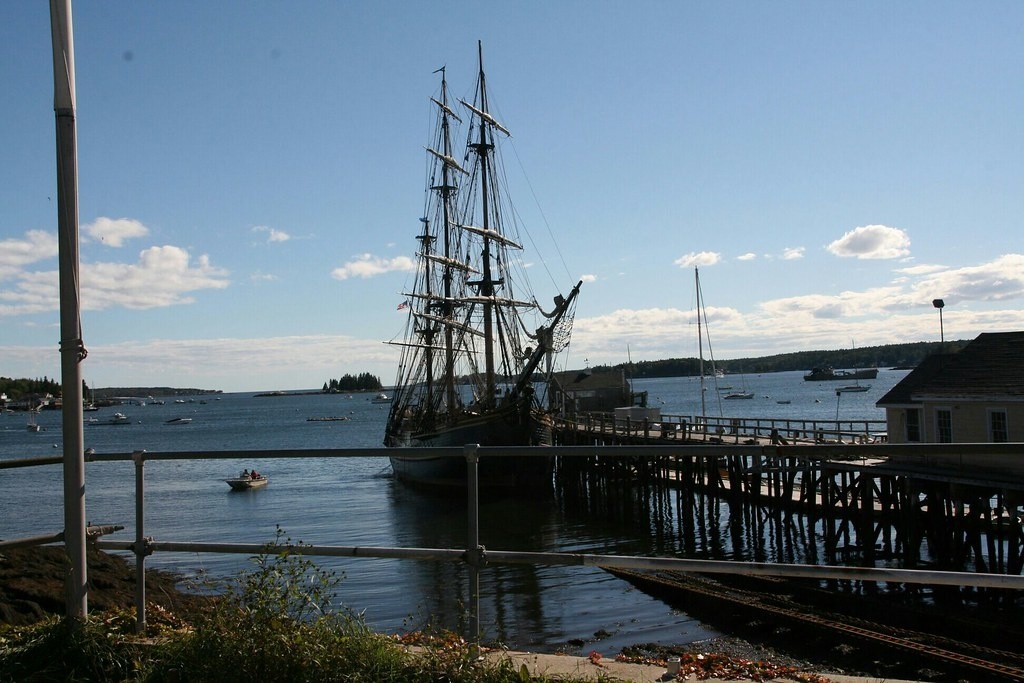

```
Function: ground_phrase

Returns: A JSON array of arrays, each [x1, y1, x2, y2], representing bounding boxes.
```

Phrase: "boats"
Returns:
[[87, 413, 132, 426], [345, 394, 352, 400], [777, 400, 791, 404], [200, 400, 208, 404], [175, 399, 186, 404], [226, 476, 268, 487], [163, 418, 192, 425], [214, 398, 220, 401], [371, 394, 392, 403], [306, 417, 351, 421], [651, 421, 702, 432]]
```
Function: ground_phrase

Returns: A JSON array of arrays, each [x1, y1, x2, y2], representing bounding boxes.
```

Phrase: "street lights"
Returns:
[[932, 299, 945, 353]]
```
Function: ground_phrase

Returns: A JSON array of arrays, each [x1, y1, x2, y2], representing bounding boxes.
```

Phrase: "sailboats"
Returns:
[[26, 402, 40, 432], [716, 369, 733, 390], [661, 266, 730, 470], [835, 339, 872, 393], [384, 37, 582, 493], [724, 363, 755, 400], [83, 381, 98, 411]]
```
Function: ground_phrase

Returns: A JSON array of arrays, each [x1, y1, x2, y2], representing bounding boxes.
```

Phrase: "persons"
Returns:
[[243, 469, 258, 478]]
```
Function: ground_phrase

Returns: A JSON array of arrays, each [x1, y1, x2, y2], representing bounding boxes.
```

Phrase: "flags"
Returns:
[[396, 299, 409, 310]]
